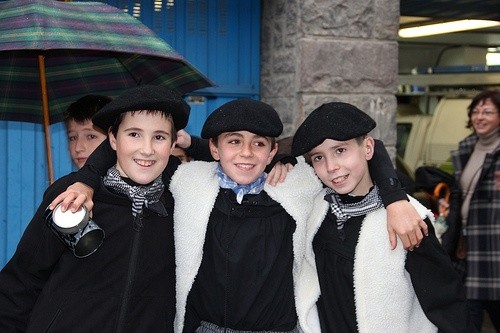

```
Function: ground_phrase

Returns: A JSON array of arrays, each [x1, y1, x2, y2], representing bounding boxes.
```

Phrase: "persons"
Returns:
[[42, 95, 113, 198], [174, 102, 473, 333], [49, 99, 428, 333], [448, 91, 500, 333], [0, 86, 297, 333]]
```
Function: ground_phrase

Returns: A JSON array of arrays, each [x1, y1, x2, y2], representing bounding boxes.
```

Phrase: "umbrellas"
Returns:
[[0, 0, 219, 183]]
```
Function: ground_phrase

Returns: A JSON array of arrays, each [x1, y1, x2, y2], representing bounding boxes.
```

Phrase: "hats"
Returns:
[[200, 98, 284, 139], [91, 84, 191, 133], [291, 102, 376, 159]]
